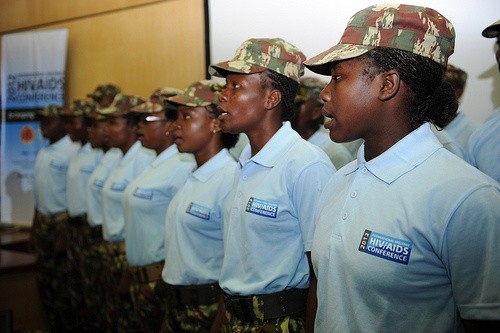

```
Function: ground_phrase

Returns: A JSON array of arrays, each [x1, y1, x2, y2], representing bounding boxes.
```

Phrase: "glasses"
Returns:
[[137, 116, 167, 123]]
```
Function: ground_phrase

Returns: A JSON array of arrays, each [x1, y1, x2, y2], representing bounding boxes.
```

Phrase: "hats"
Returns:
[[209, 37, 306, 84], [129, 87, 183, 113], [96, 93, 146, 116], [162, 79, 223, 106], [303, 2, 455, 75], [35, 83, 121, 119], [294, 76, 328, 102], [443, 65, 468, 89], [481, 19, 500, 38]]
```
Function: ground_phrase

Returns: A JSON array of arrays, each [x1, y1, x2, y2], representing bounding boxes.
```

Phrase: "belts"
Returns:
[[126, 263, 164, 284], [160, 276, 221, 306], [222, 289, 304, 321], [39, 211, 126, 254]]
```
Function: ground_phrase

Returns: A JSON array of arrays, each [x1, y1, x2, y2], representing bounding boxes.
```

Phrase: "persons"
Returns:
[[95, 93, 159, 333], [149, 80, 243, 333], [113, 87, 197, 333], [302, 4, 500, 333], [289, 20, 500, 185], [30, 84, 123, 333], [207, 37, 336, 333]]
